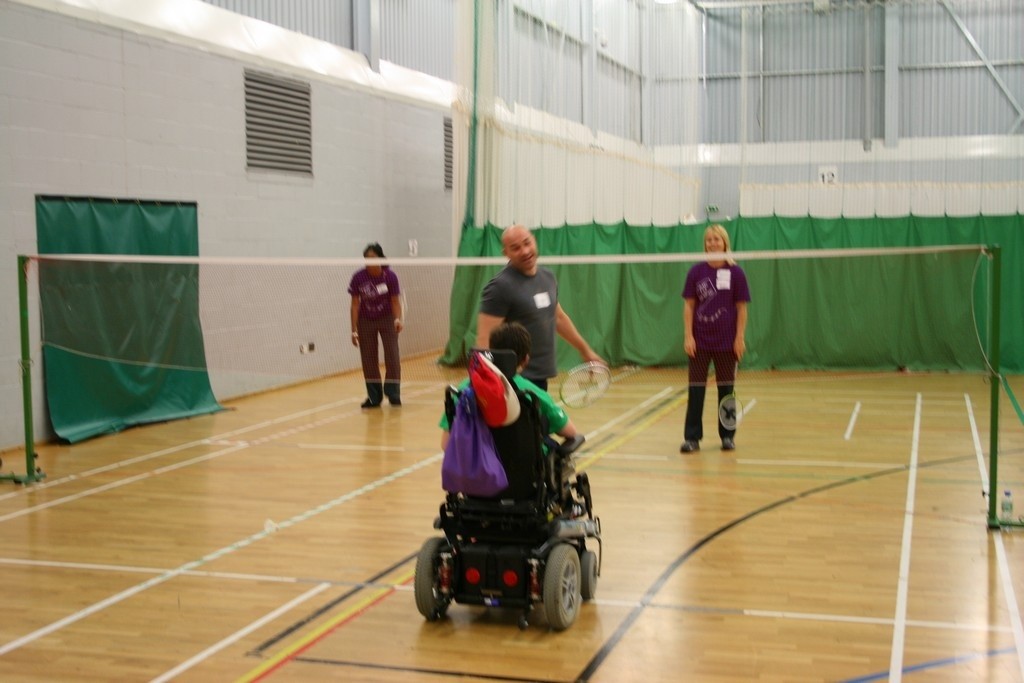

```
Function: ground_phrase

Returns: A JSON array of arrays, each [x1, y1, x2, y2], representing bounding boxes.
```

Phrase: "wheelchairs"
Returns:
[[415, 348, 601, 631]]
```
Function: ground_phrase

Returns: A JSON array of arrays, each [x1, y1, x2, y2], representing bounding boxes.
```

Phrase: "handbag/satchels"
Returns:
[[442, 387, 508, 496]]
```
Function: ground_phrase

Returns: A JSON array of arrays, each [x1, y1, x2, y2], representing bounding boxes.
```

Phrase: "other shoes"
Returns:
[[361, 395, 382, 408], [722, 435, 734, 448], [383, 390, 402, 405], [680, 440, 699, 452]]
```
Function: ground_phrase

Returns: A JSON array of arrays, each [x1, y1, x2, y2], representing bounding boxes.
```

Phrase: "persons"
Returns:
[[476, 224, 608, 394], [438, 325, 575, 456], [348, 243, 405, 409], [680, 225, 751, 451]]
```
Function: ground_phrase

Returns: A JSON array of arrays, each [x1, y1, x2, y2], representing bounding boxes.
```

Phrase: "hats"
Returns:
[[469, 351, 520, 426]]
